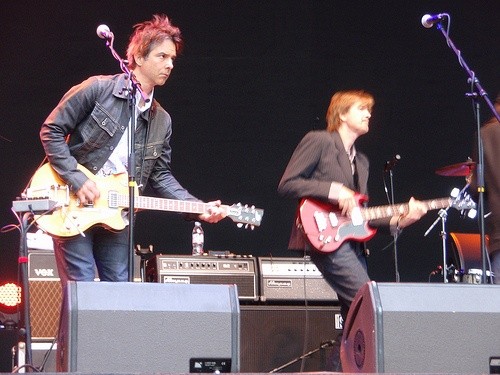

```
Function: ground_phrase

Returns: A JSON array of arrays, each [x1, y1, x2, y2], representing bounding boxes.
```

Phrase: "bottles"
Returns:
[[192, 222, 204, 257]]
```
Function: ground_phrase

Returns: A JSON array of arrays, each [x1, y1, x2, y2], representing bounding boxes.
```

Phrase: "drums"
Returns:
[[446, 233, 489, 273]]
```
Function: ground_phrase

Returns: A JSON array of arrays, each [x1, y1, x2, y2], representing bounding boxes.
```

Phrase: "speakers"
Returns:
[[339, 281, 500, 375], [26, 251, 64, 342], [146, 254, 344, 372], [54, 280, 241, 373]]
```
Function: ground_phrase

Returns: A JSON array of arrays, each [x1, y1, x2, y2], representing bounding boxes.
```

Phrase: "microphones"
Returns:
[[421, 13, 443, 28], [383, 154, 401, 173], [96, 24, 114, 39]]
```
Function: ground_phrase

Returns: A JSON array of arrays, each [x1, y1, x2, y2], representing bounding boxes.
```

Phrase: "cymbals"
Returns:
[[435, 162, 478, 177]]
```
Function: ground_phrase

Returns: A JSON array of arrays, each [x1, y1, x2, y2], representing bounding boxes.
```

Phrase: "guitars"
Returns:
[[297, 192, 477, 254], [29, 162, 264, 242]]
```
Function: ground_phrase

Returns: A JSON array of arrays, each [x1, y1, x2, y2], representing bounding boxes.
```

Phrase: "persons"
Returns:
[[25, 15, 226, 294], [278, 91, 426, 320], [471, 94, 500, 284]]
